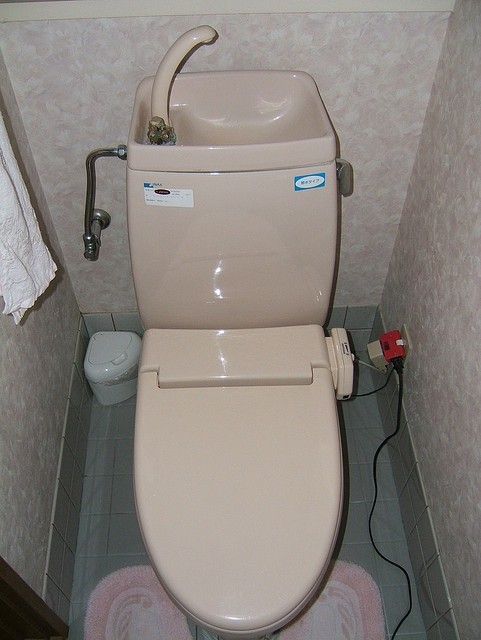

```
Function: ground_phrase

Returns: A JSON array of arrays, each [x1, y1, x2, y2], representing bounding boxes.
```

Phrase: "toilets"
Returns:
[[126, 26, 351, 640]]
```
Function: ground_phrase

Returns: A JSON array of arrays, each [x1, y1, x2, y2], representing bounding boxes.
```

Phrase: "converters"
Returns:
[[380, 330, 406, 363], [367, 340, 390, 369]]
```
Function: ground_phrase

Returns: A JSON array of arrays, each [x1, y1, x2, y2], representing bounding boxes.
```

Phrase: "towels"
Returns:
[[0, 111, 57, 327]]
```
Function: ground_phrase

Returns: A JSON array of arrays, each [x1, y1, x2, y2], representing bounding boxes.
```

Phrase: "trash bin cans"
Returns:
[[83, 331, 143, 406]]
